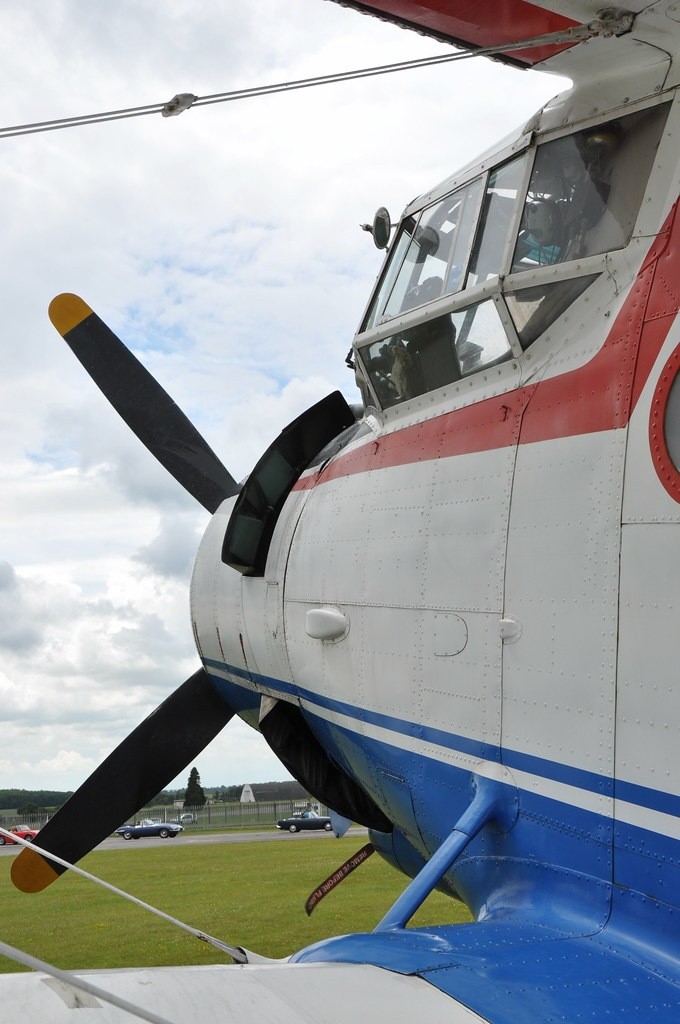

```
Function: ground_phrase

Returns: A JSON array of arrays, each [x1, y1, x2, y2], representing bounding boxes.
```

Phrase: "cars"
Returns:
[[277, 803, 333, 833], [113, 805, 197, 840], [0, 825, 40, 845]]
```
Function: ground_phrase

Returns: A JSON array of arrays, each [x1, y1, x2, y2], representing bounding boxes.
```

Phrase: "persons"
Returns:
[[301, 813, 306, 818], [9, 826, 16, 832]]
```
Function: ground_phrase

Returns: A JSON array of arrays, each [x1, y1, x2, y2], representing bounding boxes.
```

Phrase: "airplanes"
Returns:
[[0, 0, 680, 1024]]
